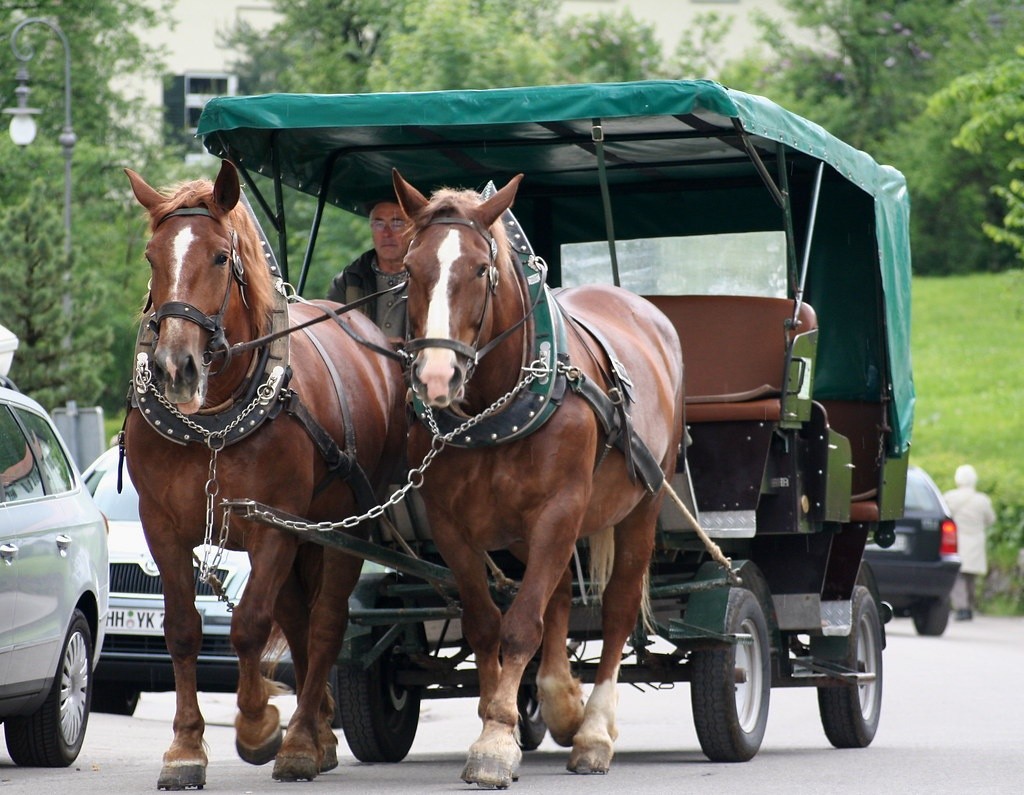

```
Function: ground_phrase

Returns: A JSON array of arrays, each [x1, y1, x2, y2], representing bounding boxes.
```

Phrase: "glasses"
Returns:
[[369, 219, 407, 233]]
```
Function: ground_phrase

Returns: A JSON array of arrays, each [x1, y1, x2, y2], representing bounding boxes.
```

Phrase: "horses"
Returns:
[[124, 162, 407, 790], [386, 165, 684, 792]]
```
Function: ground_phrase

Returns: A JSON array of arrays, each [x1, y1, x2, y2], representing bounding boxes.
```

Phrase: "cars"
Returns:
[[857, 465, 961, 638], [80, 437, 316, 714], [0, 370, 113, 767]]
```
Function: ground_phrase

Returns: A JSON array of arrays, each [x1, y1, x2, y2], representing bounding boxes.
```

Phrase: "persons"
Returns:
[[944, 466, 995, 622], [327, 198, 411, 343]]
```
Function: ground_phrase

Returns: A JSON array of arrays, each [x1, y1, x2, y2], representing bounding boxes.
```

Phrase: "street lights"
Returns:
[[2, 16, 77, 416]]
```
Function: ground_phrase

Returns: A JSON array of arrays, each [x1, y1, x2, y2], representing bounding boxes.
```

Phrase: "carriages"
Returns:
[[122, 77, 919, 767]]
